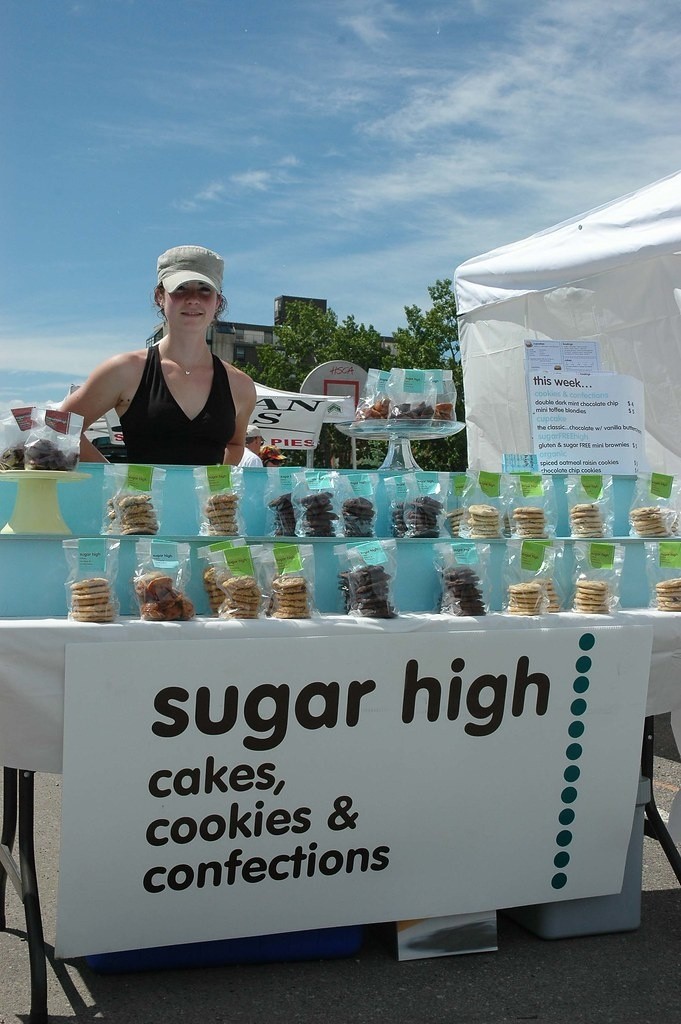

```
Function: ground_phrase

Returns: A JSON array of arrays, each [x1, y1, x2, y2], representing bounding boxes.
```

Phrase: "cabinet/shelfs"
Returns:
[[0, 460, 681, 1024]]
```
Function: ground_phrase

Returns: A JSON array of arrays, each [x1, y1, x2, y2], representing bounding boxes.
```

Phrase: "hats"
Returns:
[[256, 443, 288, 463], [157, 245, 225, 296], [245, 425, 266, 441]]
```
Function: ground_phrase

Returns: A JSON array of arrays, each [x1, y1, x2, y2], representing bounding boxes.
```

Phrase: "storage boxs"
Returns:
[[83, 920, 364, 971], [524, 774, 651, 940]]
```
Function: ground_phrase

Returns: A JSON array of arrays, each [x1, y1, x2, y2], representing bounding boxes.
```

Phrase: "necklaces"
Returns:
[[175, 357, 203, 375]]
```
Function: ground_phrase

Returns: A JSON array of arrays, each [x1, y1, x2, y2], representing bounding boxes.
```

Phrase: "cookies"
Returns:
[[69, 493, 681, 621]]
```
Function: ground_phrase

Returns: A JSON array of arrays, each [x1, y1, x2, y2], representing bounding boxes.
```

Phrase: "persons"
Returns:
[[259, 445, 286, 468], [60, 245, 257, 466], [239, 425, 263, 467]]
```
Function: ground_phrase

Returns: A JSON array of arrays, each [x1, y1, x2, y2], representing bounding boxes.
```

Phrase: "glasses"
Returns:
[[268, 459, 283, 465]]
